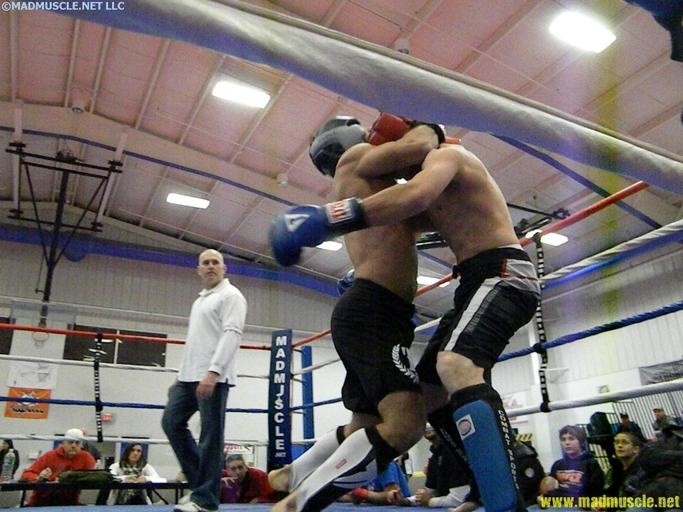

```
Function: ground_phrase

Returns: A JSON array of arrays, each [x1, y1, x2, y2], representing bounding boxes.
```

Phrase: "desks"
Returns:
[[0, 479, 195, 507]]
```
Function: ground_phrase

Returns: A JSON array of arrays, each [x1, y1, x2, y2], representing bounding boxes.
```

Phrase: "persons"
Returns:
[[268, 114, 447, 511], [268, 142, 541, 512], [0, 438, 20, 480], [219, 453, 276, 504], [162, 248, 248, 512], [22, 428, 96, 508], [106, 443, 167, 505], [337, 406, 683, 512]]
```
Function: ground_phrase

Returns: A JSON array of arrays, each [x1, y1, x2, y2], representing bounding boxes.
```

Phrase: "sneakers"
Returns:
[[174, 490, 218, 512]]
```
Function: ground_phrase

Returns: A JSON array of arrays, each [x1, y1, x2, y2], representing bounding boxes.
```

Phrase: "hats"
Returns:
[[65, 428, 83, 438]]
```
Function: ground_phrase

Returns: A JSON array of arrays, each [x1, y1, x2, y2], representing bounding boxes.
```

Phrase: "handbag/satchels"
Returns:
[[59, 468, 113, 484]]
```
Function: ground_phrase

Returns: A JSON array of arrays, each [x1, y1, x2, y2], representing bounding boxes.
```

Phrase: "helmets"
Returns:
[[308, 116, 370, 178]]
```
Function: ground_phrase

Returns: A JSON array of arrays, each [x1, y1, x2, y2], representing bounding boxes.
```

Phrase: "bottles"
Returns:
[[95, 460, 101, 469], [0, 449, 15, 483]]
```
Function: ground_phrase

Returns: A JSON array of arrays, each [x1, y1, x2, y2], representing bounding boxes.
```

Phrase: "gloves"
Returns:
[[337, 267, 354, 295], [370, 112, 409, 145], [270, 196, 365, 266]]
